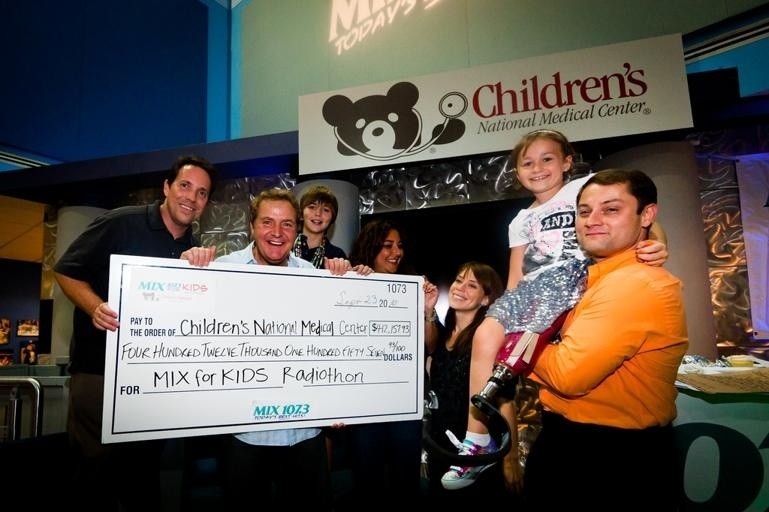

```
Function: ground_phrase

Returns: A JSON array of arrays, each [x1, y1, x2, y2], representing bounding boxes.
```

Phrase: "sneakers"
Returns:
[[440, 437, 500, 490]]
